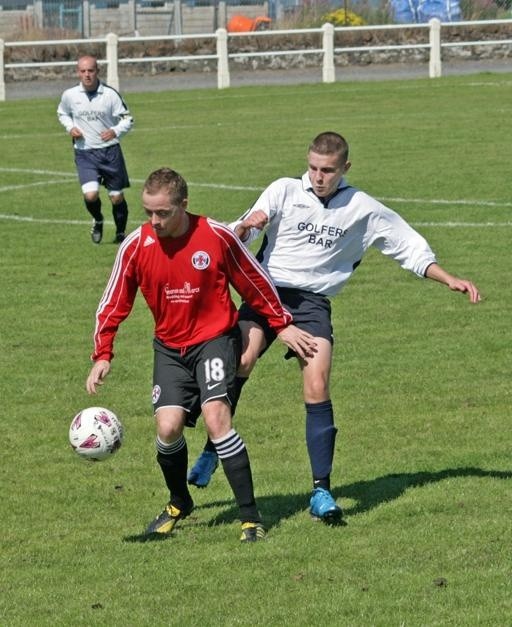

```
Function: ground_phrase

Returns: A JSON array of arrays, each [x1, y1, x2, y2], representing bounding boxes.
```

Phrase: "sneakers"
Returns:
[[240, 522, 268, 543], [113, 233, 125, 242], [189, 451, 219, 488], [90, 219, 103, 243], [309, 487, 343, 523], [146, 500, 194, 536]]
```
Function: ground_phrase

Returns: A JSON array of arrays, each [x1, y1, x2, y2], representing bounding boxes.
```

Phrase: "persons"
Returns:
[[56, 55, 134, 243], [85, 167, 319, 543], [186, 132, 482, 524]]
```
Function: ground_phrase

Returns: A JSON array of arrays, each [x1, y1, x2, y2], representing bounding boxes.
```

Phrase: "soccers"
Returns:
[[68, 407, 123, 461]]
